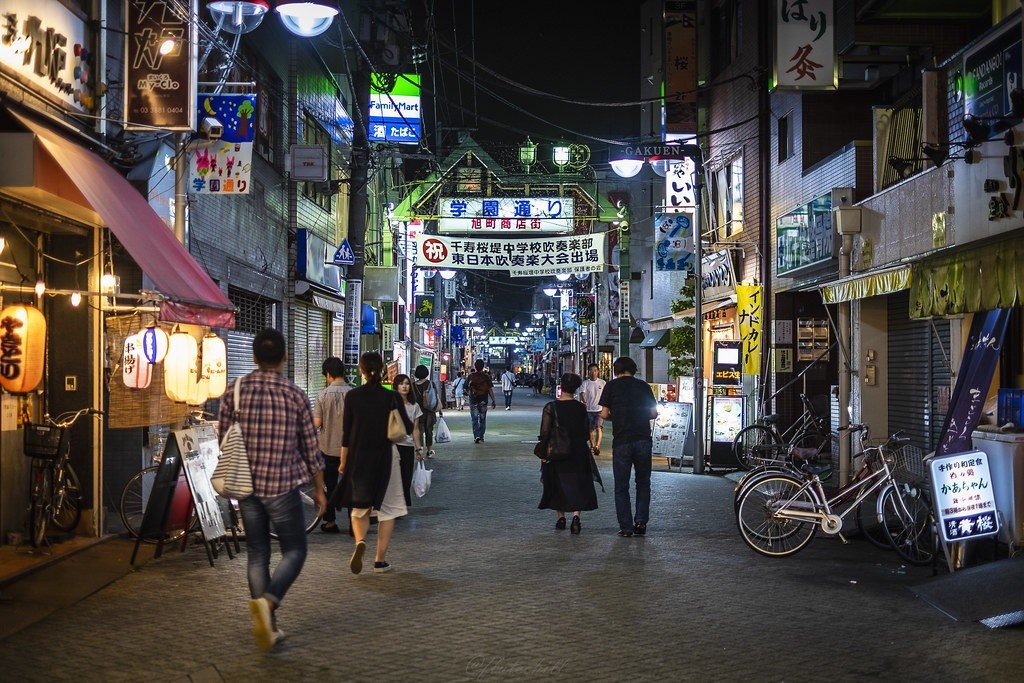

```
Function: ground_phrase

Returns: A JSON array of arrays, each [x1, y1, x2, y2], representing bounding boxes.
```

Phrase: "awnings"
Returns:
[[693, 300, 735, 317], [639, 330, 670, 349], [0, 107, 240, 330], [797, 263, 956, 375]]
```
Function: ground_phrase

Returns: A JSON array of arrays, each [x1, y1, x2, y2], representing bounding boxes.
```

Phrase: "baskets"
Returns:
[[872, 445, 924, 485], [23, 422, 61, 459]]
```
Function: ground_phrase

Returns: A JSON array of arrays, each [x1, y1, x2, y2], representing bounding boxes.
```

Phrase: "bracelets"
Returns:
[[415, 449, 422, 451]]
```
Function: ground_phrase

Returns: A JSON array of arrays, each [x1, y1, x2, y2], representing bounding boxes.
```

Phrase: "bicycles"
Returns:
[[21, 408, 107, 557], [119, 410, 330, 543], [732, 394, 944, 566]]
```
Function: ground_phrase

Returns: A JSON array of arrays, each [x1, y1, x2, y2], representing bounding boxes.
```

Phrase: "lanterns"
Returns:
[[0, 302, 46, 397], [143, 326, 169, 364], [164, 332, 226, 409], [123, 335, 153, 392]]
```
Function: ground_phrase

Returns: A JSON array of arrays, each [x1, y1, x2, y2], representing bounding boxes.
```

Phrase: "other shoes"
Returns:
[[591, 447, 600, 456], [350, 540, 366, 574], [321, 523, 339, 533], [272, 629, 286, 644], [249, 597, 274, 651], [505, 406, 510, 410], [475, 436, 480, 443], [634, 522, 646, 535], [373, 560, 392, 572], [617, 526, 634, 536]]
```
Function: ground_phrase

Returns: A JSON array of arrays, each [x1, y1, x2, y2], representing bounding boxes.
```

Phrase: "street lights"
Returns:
[[606, 145, 709, 475], [205, 1, 338, 384]]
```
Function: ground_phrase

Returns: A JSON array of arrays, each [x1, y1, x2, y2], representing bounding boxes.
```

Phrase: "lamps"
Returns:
[[888, 114, 1008, 178]]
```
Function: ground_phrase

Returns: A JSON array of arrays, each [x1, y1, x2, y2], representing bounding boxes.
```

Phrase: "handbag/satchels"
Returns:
[[210, 376, 254, 500], [451, 389, 455, 398], [422, 381, 436, 412], [534, 401, 570, 461], [512, 382, 516, 387], [435, 417, 451, 443], [388, 390, 408, 444], [412, 460, 433, 498]]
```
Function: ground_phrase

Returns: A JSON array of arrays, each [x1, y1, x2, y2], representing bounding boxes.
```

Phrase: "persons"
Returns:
[[412, 365, 442, 458], [497, 371, 500, 383], [501, 365, 514, 410], [338, 352, 414, 573], [579, 363, 607, 455], [463, 359, 496, 444], [218, 328, 327, 650], [520, 369, 525, 388], [392, 374, 423, 520], [534, 373, 605, 535], [452, 372, 465, 411], [313, 356, 355, 536], [598, 356, 658, 536]]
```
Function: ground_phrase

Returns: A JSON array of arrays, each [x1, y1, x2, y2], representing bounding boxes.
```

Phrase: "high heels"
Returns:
[[556, 516, 566, 529], [570, 515, 581, 535]]
